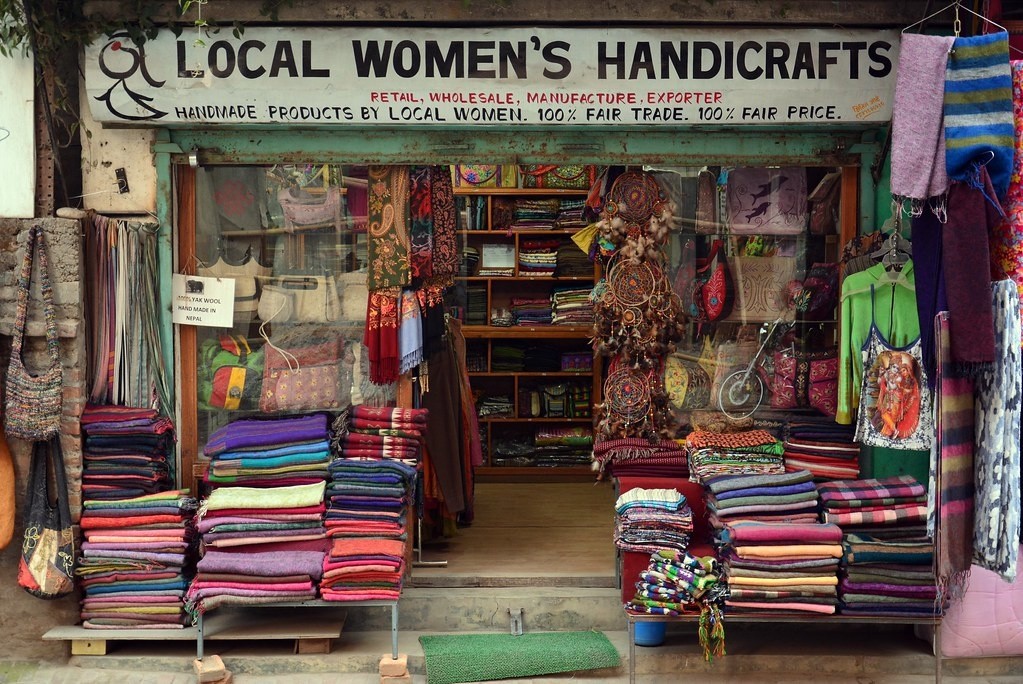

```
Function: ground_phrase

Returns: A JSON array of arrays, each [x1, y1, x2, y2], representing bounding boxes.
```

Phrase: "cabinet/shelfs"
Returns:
[[197, 218, 367, 415], [662, 233, 838, 411], [290, 164, 603, 484]]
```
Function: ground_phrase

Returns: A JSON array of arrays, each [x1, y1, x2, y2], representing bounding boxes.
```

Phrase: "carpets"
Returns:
[[418, 630, 623, 684]]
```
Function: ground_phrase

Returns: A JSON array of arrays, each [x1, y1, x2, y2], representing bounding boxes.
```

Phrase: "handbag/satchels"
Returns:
[[258, 285, 295, 324], [197, 334, 266, 413], [674, 237, 736, 324], [258, 335, 355, 451], [4, 357, 62, 442], [521, 382, 592, 419], [518, 163, 604, 191], [337, 271, 368, 323], [17, 499, 76, 599], [277, 275, 342, 324], [350, 337, 399, 407], [277, 186, 342, 233], [450, 163, 519, 190], [195, 254, 274, 312]]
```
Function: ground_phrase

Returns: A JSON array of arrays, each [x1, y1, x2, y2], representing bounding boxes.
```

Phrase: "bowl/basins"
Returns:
[[627, 621, 668, 647]]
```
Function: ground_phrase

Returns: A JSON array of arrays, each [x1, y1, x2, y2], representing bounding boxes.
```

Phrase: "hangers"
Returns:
[[67, 179, 162, 233], [990, 258, 1011, 280], [839, 199, 915, 301], [901, 0, 1023, 166]]
